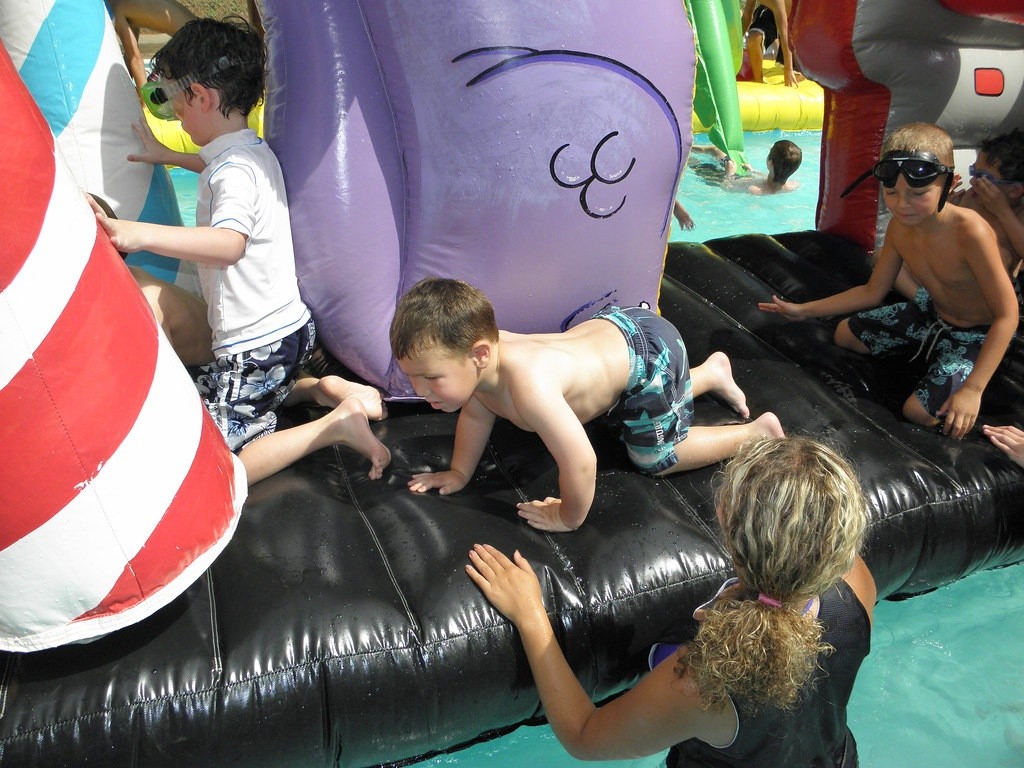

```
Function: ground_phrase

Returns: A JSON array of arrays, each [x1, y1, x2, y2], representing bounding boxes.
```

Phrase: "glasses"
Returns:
[[139, 62, 221, 121], [874, 150, 954, 188], [968, 163, 1016, 185]]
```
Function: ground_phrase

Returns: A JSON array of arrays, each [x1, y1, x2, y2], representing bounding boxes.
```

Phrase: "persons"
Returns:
[[690, 127, 801, 196], [981, 421, 1024, 469], [460, 433, 876, 768], [82, 17, 393, 491], [867, 127, 1024, 340], [754, 120, 1020, 443], [671, 198, 694, 232], [389, 275, 787, 531], [106, 1, 199, 111], [741, 0, 802, 88]]
